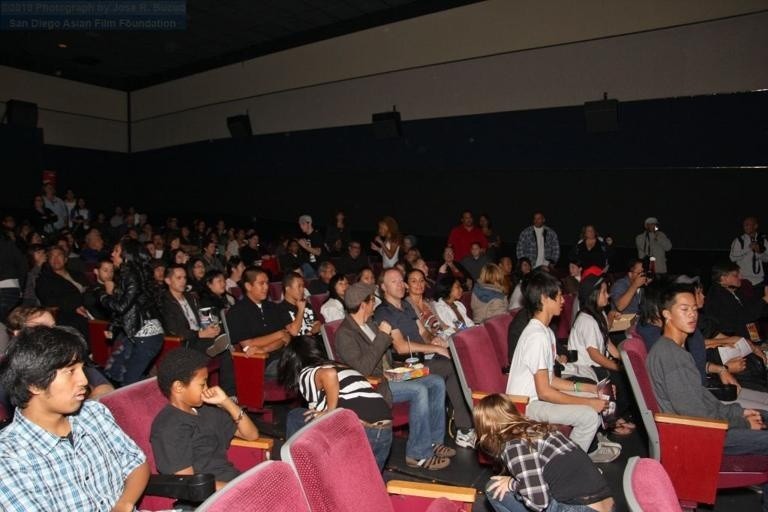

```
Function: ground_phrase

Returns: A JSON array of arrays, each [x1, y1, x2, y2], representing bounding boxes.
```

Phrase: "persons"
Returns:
[[636, 216, 672, 279], [395, 259, 412, 279], [635, 279, 768, 411], [149, 347, 259, 493], [335, 282, 456, 471], [472, 262, 510, 324], [405, 268, 453, 347], [509, 265, 553, 313], [277, 335, 392, 474], [506, 270, 622, 464], [438, 246, 470, 279], [279, 272, 322, 336], [576, 224, 607, 267], [1, 170, 154, 326], [729, 216, 768, 286], [326, 210, 353, 255], [225, 266, 291, 378], [432, 275, 476, 338], [346, 240, 368, 270], [357, 267, 382, 313], [472, 393, 615, 512], [610, 257, 652, 315], [413, 258, 437, 289], [288, 215, 324, 264], [0, 325, 184, 512], [516, 211, 560, 269], [226, 224, 280, 268], [310, 260, 336, 294], [478, 214, 511, 257], [371, 216, 399, 269], [507, 272, 614, 444], [675, 274, 767, 350], [448, 210, 487, 262], [604, 234, 615, 247], [706, 261, 768, 323], [403, 235, 416, 253], [564, 259, 585, 292], [462, 241, 491, 280], [320, 274, 351, 324], [282, 241, 304, 272], [568, 273, 637, 436], [373, 267, 479, 450], [9, 304, 115, 400], [406, 248, 428, 275], [645, 283, 768, 512], [155, 216, 226, 351], [570, 266, 626, 344]]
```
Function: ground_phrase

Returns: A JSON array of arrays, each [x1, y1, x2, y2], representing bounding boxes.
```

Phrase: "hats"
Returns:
[[344, 282, 376, 309], [645, 217, 660, 226], [580, 274, 605, 296], [581, 264, 609, 282]]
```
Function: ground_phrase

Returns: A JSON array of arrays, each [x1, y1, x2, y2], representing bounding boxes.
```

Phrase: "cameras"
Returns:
[[641, 271, 654, 288], [753, 235, 766, 254]]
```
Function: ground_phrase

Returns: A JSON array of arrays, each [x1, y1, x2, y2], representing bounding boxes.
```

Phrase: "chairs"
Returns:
[[484, 313, 531, 367], [192, 459, 313, 512], [269, 281, 287, 302], [624, 457, 681, 512], [281, 407, 478, 512], [447, 326, 574, 463], [99, 376, 273, 487], [227, 285, 247, 298], [320, 317, 411, 426], [220, 307, 296, 412], [307, 292, 330, 322], [617, 337, 767, 500], [261, 254, 286, 277]]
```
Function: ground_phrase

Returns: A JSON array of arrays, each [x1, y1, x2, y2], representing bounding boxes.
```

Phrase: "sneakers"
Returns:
[[597, 432, 622, 450], [206, 334, 228, 357], [455, 428, 477, 449], [431, 443, 457, 457], [405, 457, 450, 470], [588, 445, 620, 463]]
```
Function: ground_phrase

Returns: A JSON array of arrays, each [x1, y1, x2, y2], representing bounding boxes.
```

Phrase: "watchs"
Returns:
[[235, 409, 245, 424]]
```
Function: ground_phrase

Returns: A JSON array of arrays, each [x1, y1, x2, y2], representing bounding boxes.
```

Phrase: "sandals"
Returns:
[[609, 425, 631, 436], [616, 418, 636, 429]]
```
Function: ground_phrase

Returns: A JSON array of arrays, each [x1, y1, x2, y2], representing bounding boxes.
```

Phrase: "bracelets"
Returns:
[[572, 380, 581, 392]]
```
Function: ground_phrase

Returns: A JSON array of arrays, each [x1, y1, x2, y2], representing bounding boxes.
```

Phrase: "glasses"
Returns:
[[369, 297, 375, 301]]
